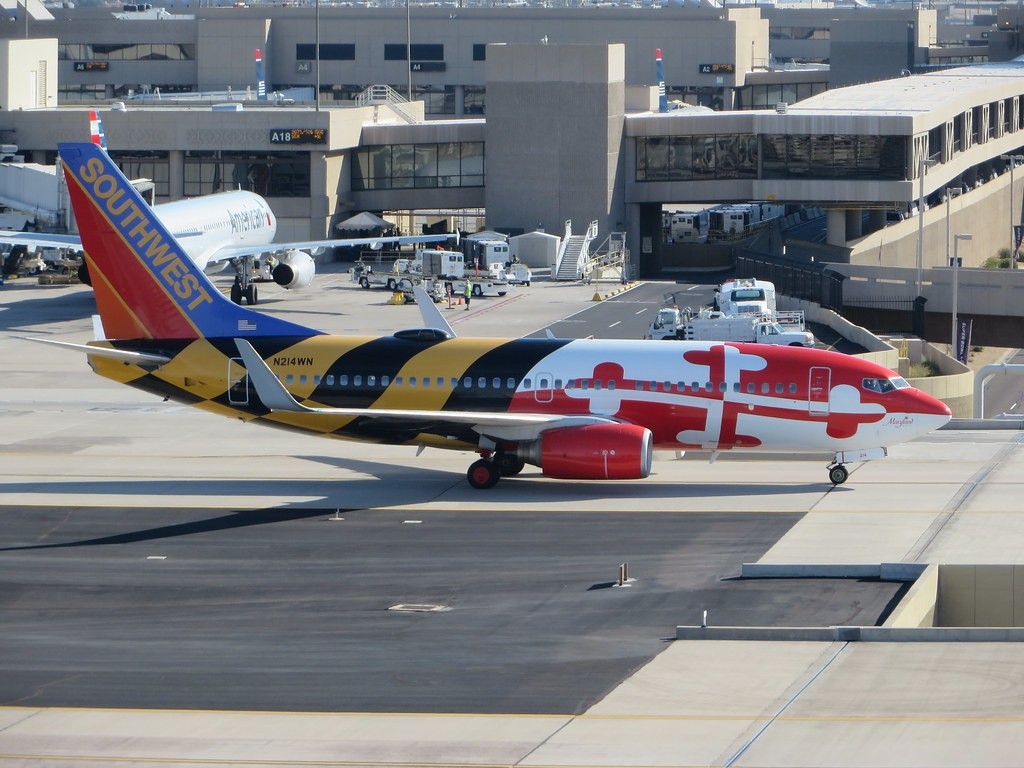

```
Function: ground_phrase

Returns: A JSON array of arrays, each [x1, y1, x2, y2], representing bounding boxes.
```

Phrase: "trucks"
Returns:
[[348, 228, 532, 304], [642, 275, 815, 348]]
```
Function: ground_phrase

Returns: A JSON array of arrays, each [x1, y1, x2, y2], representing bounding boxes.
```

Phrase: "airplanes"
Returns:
[[0, 175, 462, 307], [55, 107, 954, 491]]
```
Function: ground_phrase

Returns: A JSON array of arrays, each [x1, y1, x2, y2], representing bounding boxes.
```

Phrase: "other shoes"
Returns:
[[464, 309, 469, 310]]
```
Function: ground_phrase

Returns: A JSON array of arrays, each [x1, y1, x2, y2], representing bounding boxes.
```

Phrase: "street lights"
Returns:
[[952, 233, 974, 359], [916, 159, 937, 297], [999, 154, 1024, 270], [945, 186, 962, 267]]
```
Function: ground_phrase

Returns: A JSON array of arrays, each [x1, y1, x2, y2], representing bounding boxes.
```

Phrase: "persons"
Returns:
[[462, 279, 472, 311], [511, 254, 520, 263], [384, 227, 444, 251]]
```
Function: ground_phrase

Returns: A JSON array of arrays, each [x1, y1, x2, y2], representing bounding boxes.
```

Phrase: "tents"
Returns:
[[336, 213, 396, 254]]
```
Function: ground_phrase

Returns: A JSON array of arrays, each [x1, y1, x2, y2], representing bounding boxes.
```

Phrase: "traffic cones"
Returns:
[[457, 296, 464, 305]]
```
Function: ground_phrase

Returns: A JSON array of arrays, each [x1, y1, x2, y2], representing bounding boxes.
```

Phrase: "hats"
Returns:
[[466, 280, 470, 283]]
